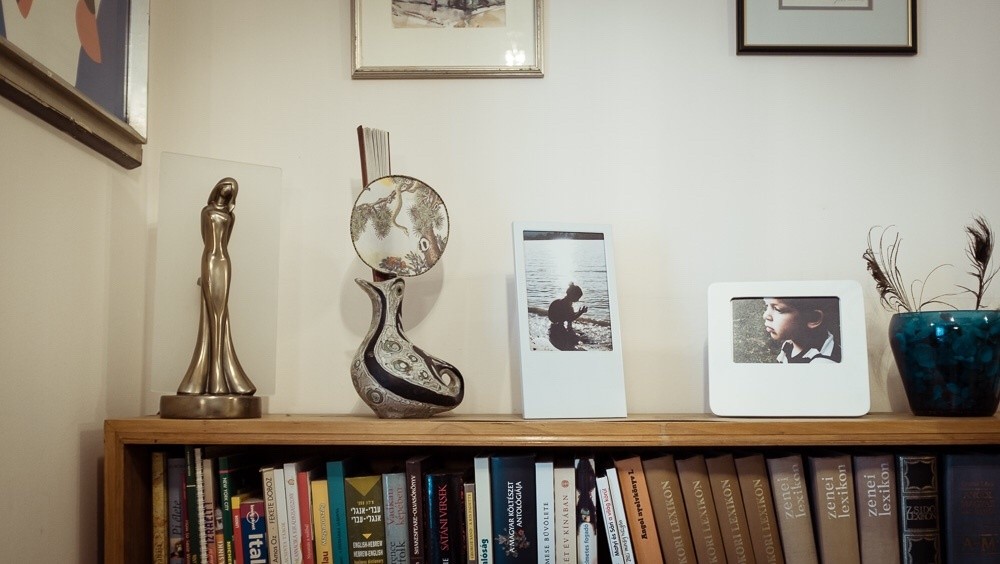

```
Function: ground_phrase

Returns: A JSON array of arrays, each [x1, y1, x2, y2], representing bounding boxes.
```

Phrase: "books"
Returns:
[[152, 447, 1000, 564]]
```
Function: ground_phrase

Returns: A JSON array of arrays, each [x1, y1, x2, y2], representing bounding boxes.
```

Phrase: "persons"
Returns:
[[547, 281, 588, 330], [175, 177, 258, 395], [763, 297, 841, 364]]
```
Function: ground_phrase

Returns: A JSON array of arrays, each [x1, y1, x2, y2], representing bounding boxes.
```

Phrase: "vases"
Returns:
[[889, 310, 1000, 417]]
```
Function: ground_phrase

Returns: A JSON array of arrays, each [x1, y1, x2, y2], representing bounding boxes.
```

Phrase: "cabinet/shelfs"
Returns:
[[102, 413, 1000, 564]]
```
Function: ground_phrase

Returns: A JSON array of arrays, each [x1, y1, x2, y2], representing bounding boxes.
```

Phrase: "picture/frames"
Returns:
[[0, 0, 149, 169], [351, 0, 544, 79], [736, 0, 918, 56], [707, 280, 871, 417]]
